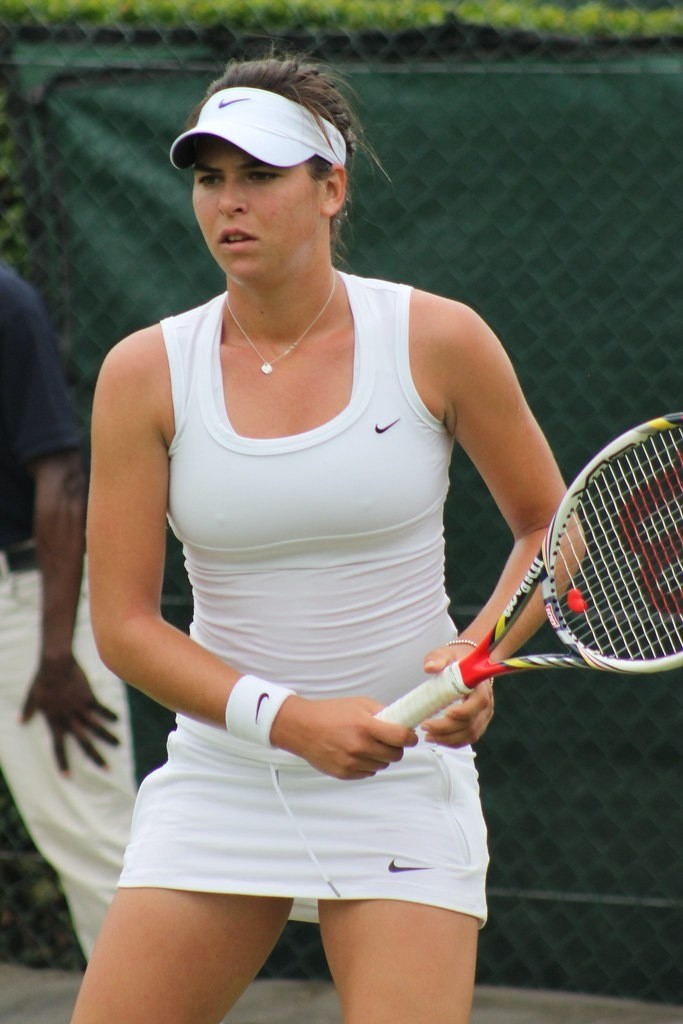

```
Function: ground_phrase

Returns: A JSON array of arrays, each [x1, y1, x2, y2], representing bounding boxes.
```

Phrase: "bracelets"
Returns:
[[224, 671, 297, 750], [444, 639, 497, 690]]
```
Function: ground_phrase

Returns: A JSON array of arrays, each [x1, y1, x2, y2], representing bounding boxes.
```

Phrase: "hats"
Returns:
[[166, 86, 352, 169]]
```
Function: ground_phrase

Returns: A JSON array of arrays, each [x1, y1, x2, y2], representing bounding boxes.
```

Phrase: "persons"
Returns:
[[66, 39, 585, 1024], [0, 267, 137, 969]]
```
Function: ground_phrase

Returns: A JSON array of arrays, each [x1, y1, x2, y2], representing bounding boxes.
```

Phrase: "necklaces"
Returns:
[[225, 264, 336, 374]]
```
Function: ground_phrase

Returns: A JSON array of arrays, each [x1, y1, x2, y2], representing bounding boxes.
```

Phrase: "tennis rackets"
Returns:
[[369, 410, 683, 730]]
